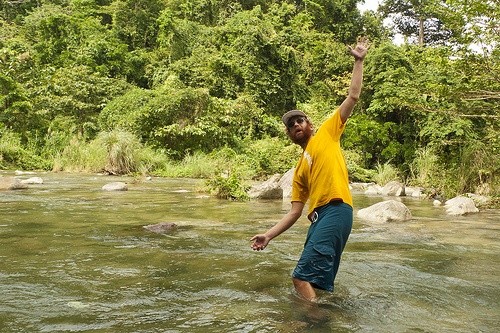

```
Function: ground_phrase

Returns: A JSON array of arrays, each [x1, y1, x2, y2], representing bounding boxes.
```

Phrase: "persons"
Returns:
[[250, 36, 372, 304]]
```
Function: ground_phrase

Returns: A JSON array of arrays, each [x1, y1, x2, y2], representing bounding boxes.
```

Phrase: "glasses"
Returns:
[[288, 118, 305, 127]]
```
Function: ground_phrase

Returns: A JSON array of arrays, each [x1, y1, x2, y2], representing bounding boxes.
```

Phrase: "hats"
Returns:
[[282, 109, 307, 126]]
